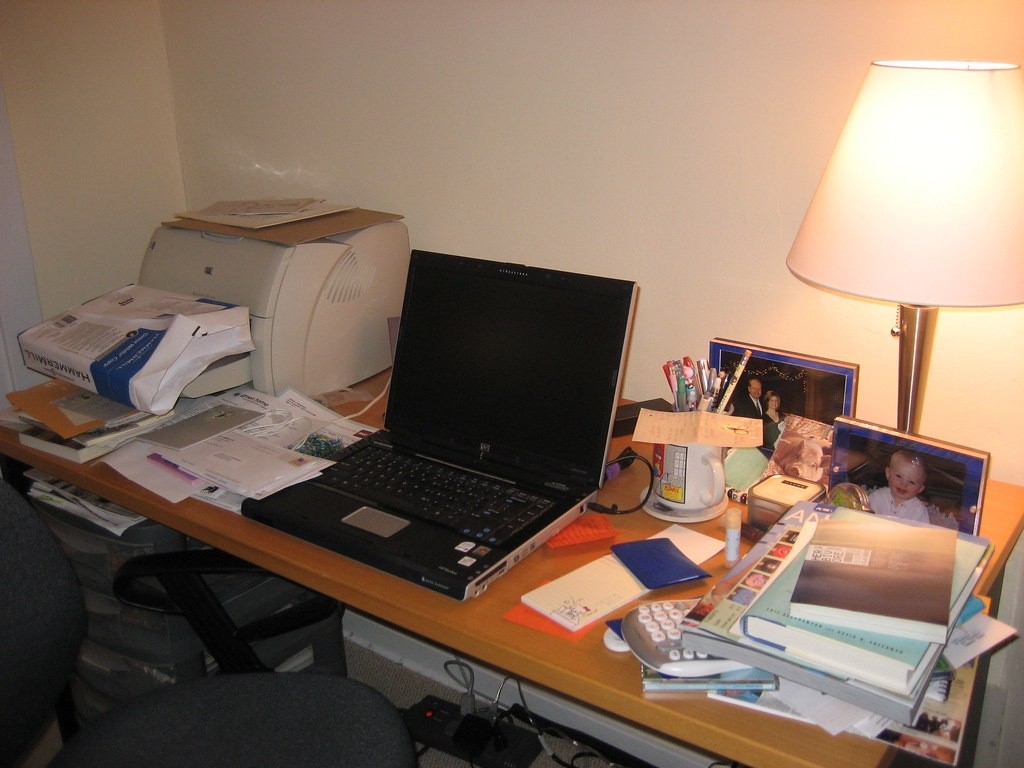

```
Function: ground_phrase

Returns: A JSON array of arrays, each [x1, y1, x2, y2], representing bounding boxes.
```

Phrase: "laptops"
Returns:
[[242, 249, 643, 600]]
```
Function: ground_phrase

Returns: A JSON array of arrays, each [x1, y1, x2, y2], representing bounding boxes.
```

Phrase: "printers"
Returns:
[[137, 217, 411, 400]]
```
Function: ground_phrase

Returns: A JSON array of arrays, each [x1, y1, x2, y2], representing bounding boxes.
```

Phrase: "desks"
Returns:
[[0, 366, 1024, 768]]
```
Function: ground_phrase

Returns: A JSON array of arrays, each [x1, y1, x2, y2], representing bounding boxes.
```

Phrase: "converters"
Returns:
[[612, 397, 673, 438]]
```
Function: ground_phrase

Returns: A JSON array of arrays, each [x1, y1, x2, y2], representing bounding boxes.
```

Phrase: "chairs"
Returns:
[[0, 478, 417, 768]]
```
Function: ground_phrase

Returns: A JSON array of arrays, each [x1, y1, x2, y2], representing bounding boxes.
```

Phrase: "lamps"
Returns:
[[786, 59, 1024, 435]]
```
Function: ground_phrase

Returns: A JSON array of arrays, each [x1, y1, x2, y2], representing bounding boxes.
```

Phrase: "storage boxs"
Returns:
[[28, 498, 308, 666], [73, 623, 346, 727]]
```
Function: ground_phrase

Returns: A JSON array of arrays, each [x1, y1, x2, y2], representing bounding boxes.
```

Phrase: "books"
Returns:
[[642, 501, 994, 728], [0, 380, 266, 464]]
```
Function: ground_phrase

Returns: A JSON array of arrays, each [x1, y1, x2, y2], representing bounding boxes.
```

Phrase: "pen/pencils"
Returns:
[[662, 350, 752, 414]]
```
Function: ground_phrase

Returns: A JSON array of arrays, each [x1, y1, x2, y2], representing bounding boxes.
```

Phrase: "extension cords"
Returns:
[[402, 694, 543, 768]]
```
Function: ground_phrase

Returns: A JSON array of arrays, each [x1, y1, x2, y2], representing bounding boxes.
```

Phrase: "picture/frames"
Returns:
[[708, 338, 860, 460], [827, 415, 990, 535]]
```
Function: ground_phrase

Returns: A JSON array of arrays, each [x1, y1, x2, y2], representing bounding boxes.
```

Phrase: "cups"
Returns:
[[652, 443, 726, 511]]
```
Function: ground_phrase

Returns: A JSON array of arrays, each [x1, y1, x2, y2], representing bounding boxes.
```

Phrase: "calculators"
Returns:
[[619, 597, 755, 680]]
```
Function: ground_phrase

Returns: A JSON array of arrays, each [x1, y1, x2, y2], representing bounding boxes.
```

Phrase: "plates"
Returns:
[[641, 485, 729, 524]]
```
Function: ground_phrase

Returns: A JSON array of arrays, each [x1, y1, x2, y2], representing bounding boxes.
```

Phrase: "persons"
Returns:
[[869, 450, 929, 524], [736, 378, 785, 450]]
